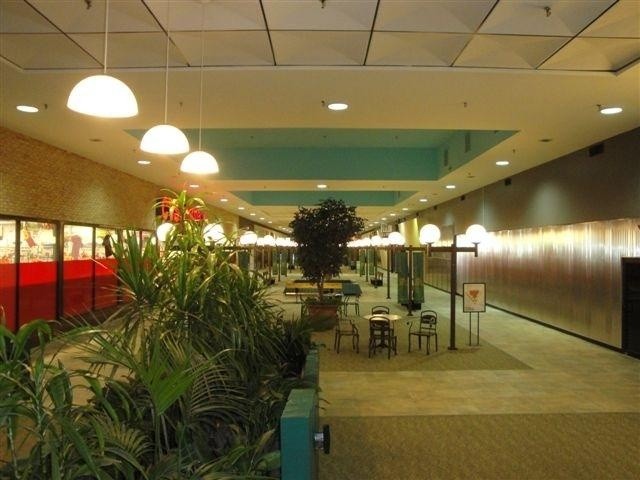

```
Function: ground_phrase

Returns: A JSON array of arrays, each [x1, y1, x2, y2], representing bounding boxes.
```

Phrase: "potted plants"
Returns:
[[288, 196, 369, 329]]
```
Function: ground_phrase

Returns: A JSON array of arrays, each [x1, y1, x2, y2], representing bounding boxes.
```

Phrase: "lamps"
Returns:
[[66, 1, 220, 176]]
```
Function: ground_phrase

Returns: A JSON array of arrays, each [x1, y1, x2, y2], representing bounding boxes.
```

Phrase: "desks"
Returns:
[[284, 281, 342, 303]]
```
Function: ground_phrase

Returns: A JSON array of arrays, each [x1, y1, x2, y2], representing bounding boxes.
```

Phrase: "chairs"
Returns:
[[333, 305, 438, 359]]
